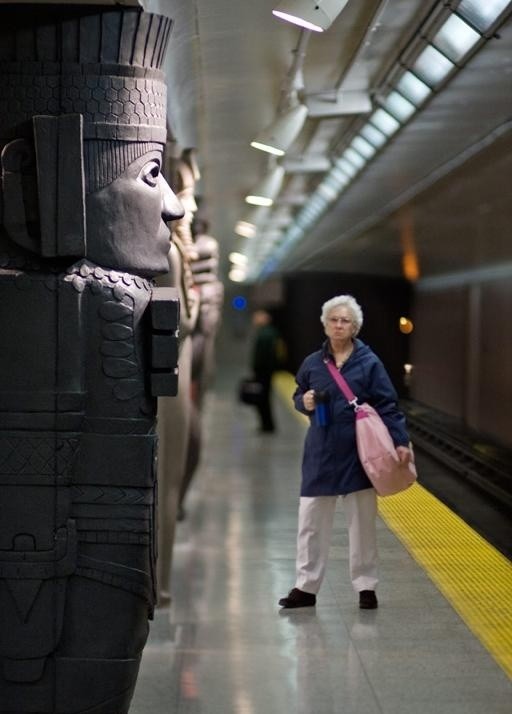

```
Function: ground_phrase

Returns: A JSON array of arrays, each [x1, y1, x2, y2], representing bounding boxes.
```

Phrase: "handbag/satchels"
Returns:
[[354, 403, 417, 497], [241, 381, 267, 403]]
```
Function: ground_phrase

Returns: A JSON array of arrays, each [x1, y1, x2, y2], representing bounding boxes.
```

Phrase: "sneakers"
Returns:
[[360, 590, 377, 608], [278, 589, 315, 607]]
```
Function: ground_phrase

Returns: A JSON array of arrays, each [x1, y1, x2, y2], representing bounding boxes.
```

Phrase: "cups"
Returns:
[[314, 391, 331, 428]]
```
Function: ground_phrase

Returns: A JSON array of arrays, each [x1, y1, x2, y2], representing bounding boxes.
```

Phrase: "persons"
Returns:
[[238, 309, 279, 434], [279, 294, 412, 609]]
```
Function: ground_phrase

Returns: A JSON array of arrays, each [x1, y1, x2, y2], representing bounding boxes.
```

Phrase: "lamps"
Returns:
[[226, 0, 349, 281]]
